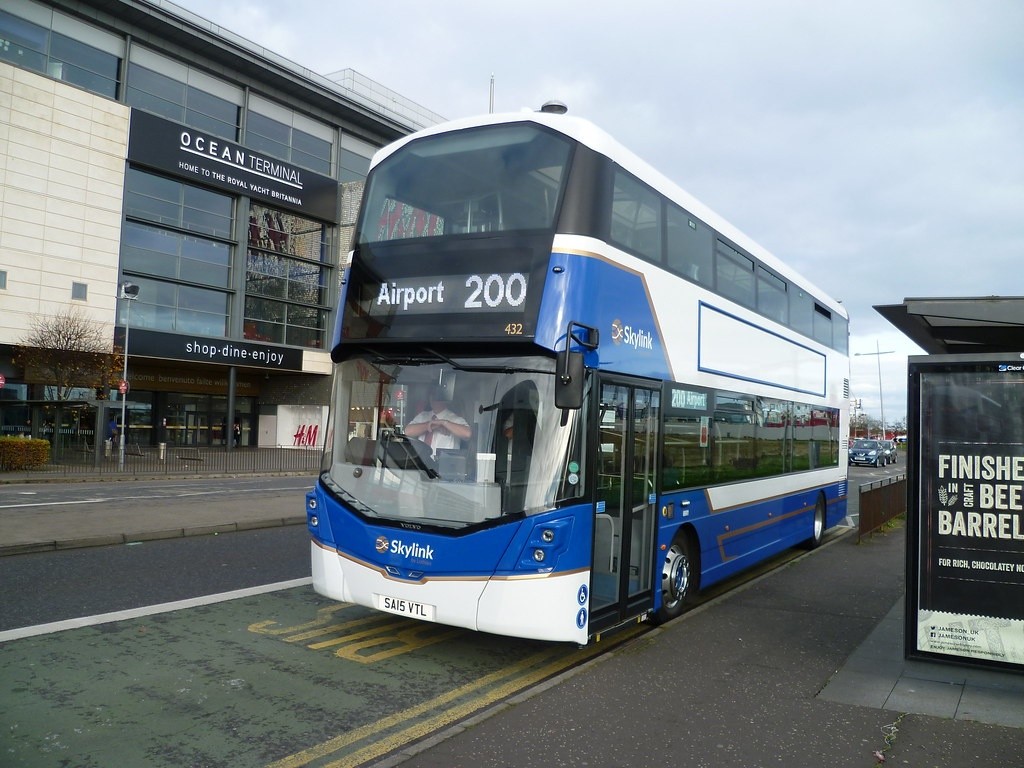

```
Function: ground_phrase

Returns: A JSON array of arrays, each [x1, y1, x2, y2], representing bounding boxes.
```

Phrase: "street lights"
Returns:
[[116, 281, 141, 472], [854, 339, 895, 441]]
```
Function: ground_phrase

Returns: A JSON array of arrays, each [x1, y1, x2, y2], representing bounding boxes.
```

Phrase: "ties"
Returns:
[[424, 415, 437, 447]]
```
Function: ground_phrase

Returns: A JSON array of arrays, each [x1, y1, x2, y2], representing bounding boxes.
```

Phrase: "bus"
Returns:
[[302, 101, 858, 648]]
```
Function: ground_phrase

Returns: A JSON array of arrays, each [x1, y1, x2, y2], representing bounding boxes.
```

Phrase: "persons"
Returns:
[[403, 386, 473, 469]]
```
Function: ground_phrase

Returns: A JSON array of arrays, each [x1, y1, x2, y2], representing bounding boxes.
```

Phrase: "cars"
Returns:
[[877, 440, 899, 464], [848, 437, 887, 468], [848, 436, 907, 451]]
